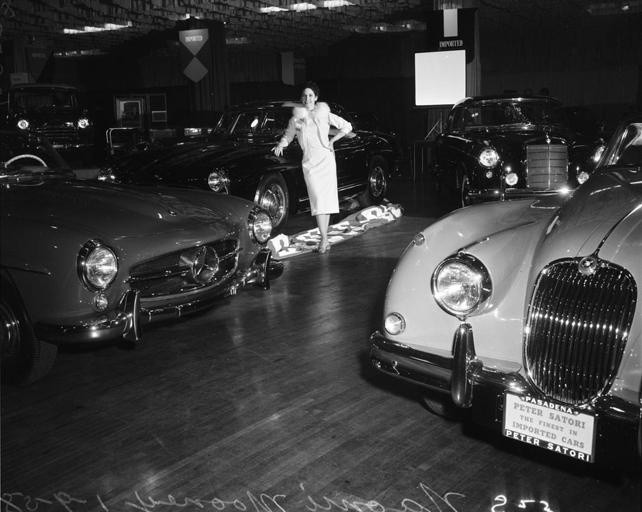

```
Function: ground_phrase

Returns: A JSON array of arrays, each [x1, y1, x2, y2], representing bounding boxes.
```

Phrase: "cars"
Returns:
[[97, 98, 406, 235], [2, 127, 274, 361]]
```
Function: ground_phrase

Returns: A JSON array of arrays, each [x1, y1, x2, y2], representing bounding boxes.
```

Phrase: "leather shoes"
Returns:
[[319, 240, 330, 253]]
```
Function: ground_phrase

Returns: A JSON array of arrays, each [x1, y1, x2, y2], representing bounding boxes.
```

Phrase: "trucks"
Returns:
[[1, 83, 96, 165]]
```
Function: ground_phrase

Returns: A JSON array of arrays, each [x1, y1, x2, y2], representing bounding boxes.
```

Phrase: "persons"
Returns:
[[270, 79, 353, 254]]
